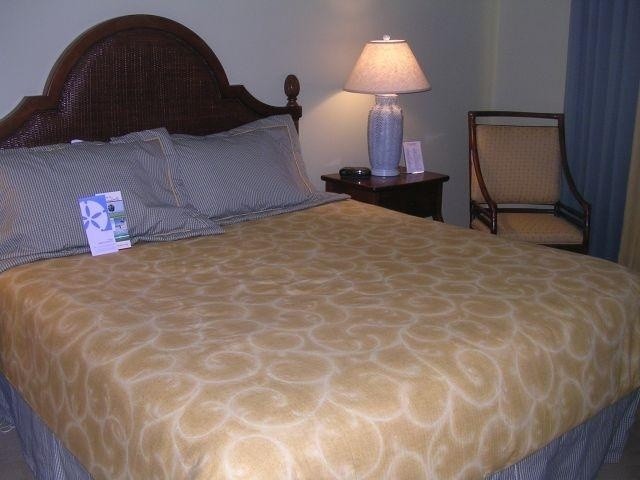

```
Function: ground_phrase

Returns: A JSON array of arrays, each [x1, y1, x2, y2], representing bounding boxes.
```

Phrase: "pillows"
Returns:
[[170, 115, 351, 225], [0, 128, 228, 274]]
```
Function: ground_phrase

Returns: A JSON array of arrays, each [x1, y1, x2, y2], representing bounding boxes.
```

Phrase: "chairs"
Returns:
[[468, 111, 591, 254]]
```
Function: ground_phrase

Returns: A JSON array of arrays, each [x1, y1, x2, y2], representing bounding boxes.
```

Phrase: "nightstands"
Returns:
[[320, 165, 449, 223]]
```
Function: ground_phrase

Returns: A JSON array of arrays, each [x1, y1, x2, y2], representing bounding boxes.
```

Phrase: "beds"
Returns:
[[0, 16, 638, 479]]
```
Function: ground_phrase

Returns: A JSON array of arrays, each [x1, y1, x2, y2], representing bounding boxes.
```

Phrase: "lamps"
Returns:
[[343, 35, 433, 177]]
